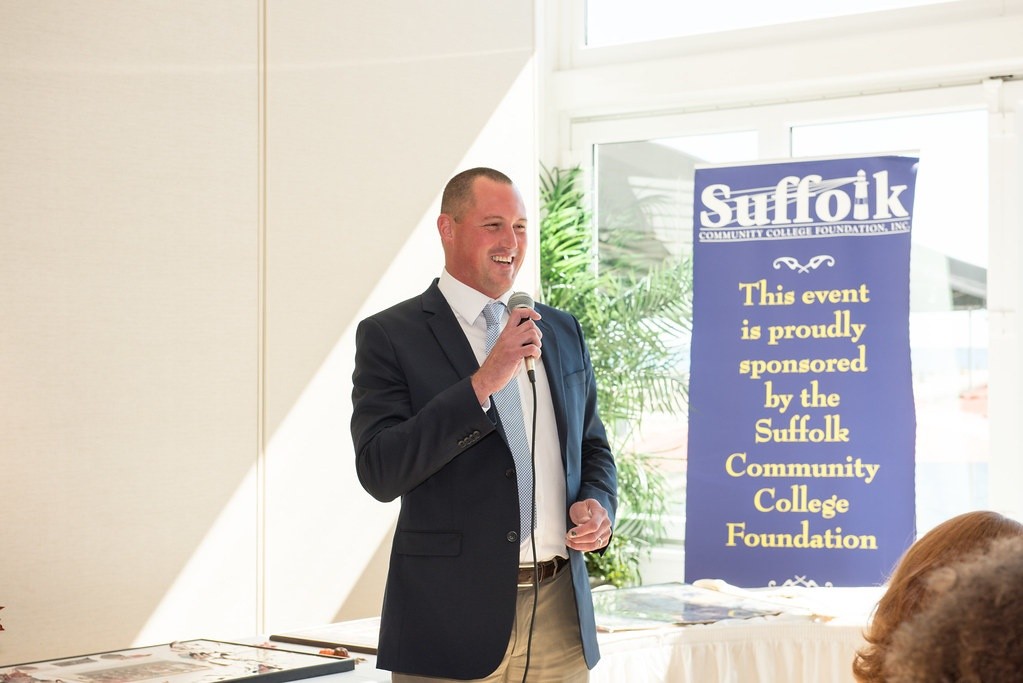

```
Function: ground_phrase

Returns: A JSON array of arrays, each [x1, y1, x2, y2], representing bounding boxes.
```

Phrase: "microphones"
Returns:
[[507, 291, 536, 383]]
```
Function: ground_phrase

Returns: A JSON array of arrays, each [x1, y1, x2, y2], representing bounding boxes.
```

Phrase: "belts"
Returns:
[[518, 556, 570, 585]]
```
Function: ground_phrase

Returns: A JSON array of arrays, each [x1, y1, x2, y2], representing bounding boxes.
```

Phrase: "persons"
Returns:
[[350, 168, 617, 683], [853, 509, 1023, 683]]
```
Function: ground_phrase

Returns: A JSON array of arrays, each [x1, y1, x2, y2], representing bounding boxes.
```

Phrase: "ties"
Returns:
[[482, 303, 537, 543]]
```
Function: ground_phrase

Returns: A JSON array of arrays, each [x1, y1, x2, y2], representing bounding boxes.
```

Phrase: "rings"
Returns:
[[598, 538, 603, 546]]
